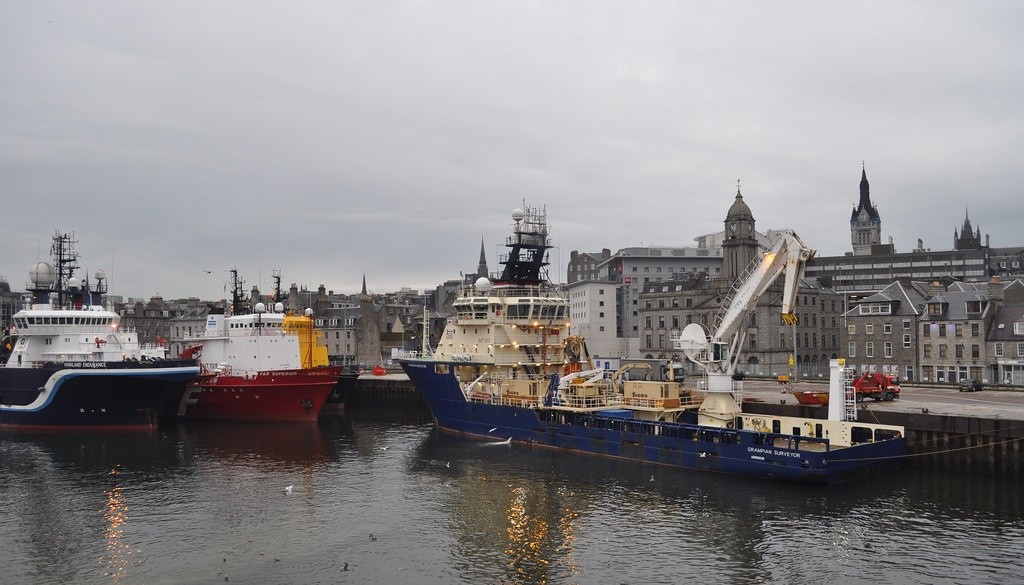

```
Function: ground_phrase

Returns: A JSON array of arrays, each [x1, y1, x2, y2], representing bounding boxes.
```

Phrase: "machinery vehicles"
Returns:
[[792, 368, 899, 404]]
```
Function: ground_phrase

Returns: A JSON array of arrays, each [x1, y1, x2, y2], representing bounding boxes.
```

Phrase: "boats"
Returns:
[[176, 268, 345, 421], [397, 203, 911, 486], [0, 229, 199, 439]]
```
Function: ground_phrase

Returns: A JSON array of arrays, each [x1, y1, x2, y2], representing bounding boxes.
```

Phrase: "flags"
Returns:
[[269, 293, 277, 301], [158, 336, 168, 342]]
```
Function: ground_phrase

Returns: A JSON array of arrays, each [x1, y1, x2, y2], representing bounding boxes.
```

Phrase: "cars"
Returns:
[[960, 378, 983, 393]]
